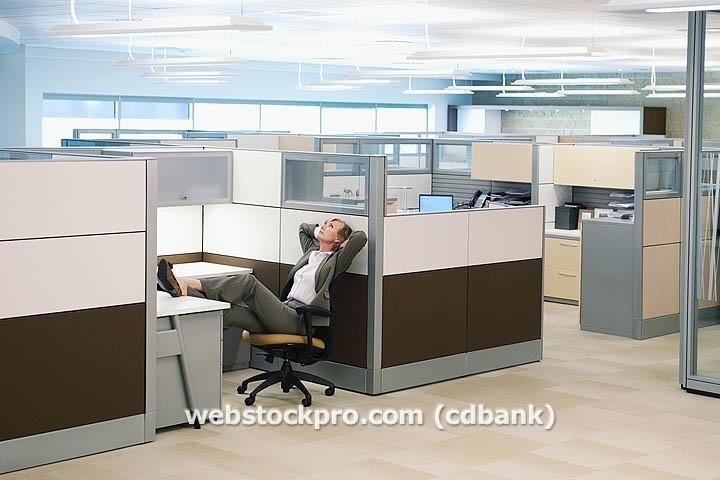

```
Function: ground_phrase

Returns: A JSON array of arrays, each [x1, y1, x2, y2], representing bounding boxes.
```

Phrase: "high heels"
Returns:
[[157, 258, 182, 297]]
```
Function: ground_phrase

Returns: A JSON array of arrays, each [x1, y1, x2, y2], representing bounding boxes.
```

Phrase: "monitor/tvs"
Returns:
[[419, 193, 453, 212]]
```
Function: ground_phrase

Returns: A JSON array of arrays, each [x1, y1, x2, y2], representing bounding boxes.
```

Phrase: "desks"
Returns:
[[154, 260, 255, 429]]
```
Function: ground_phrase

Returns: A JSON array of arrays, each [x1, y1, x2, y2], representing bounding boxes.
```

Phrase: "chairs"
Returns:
[[237, 280, 337, 408]]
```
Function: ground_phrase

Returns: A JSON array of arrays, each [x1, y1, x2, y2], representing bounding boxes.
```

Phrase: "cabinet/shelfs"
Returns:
[[470, 141, 661, 191], [545, 225, 581, 306]]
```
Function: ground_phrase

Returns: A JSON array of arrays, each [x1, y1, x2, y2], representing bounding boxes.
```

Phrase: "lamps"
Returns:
[[45, 1, 720, 100]]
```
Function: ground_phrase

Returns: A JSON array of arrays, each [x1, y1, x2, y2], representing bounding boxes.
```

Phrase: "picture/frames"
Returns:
[[579, 207, 614, 231]]
